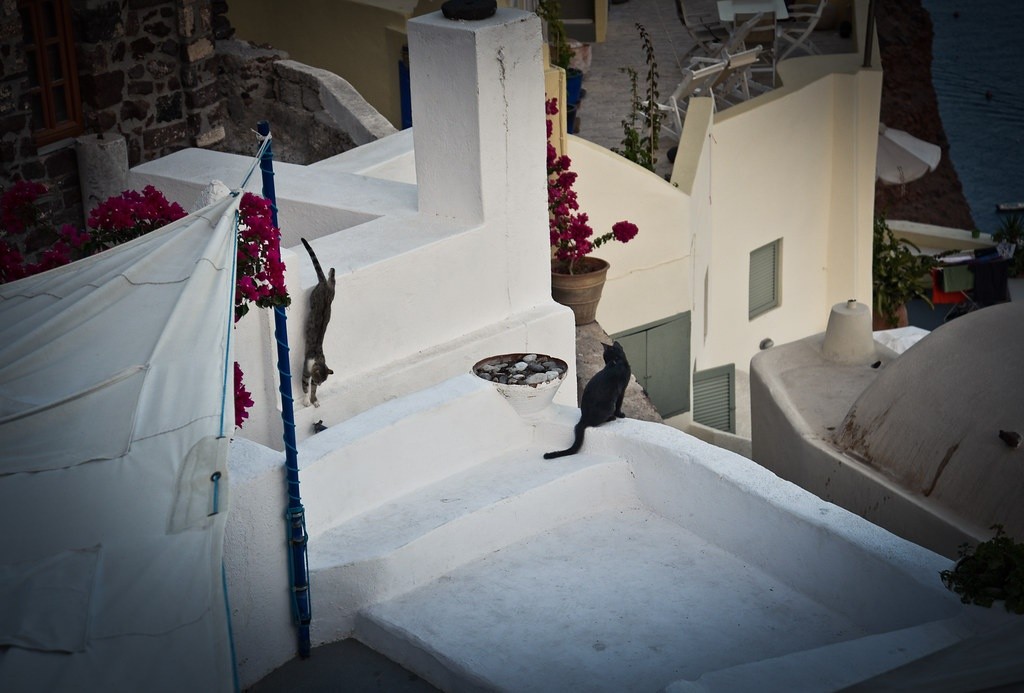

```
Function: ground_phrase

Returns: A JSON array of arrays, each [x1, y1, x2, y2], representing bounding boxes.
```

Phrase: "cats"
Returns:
[[299, 237, 336, 407], [543, 339, 633, 460]]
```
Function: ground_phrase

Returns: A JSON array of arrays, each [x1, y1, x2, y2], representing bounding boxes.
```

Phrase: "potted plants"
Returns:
[[873, 222, 960, 330], [535, 0, 583, 108], [991, 212, 1024, 278]]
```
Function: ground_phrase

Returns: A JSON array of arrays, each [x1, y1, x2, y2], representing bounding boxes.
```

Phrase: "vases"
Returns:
[[567, 103, 577, 134], [551, 257, 610, 325]]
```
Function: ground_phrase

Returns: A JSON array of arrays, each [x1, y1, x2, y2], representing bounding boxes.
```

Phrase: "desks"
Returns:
[[716, 0, 789, 39]]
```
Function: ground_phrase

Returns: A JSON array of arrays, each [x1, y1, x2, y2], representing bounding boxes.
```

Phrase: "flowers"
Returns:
[[234, 191, 291, 428], [546, 97, 639, 275], [87, 185, 189, 253], [0, 179, 91, 285]]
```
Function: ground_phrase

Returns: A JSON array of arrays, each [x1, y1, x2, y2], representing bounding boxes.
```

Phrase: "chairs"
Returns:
[[677, 45, 763, 110], [734, 11, 777, 89], [637, 59, 728, 142], [675, 0, 734, 69], [769, 0, 828, 61], [693, 11, 772, 101]]
